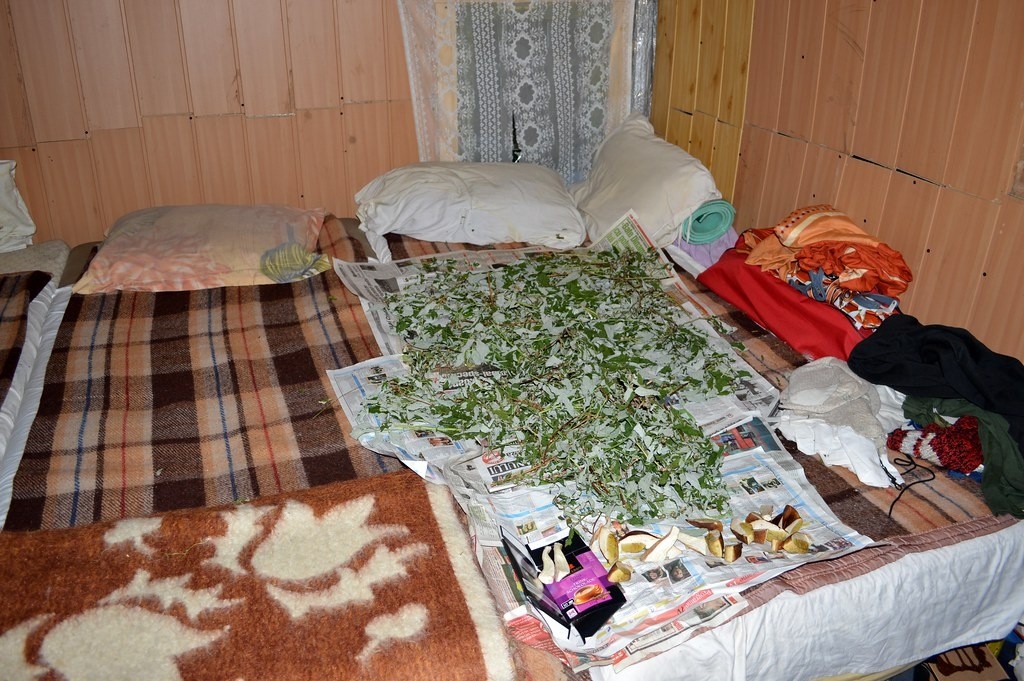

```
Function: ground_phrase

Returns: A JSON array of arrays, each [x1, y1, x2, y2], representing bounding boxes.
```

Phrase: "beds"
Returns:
[[0, 219, 1024, 681]]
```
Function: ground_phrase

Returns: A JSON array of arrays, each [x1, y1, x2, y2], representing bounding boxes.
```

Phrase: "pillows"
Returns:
[[71, 199, 332, 295], [355, 161, 588, 251], [570, 112, 724, 248], [0, 157, 37, 252], [698, 227, 897, 362]]
